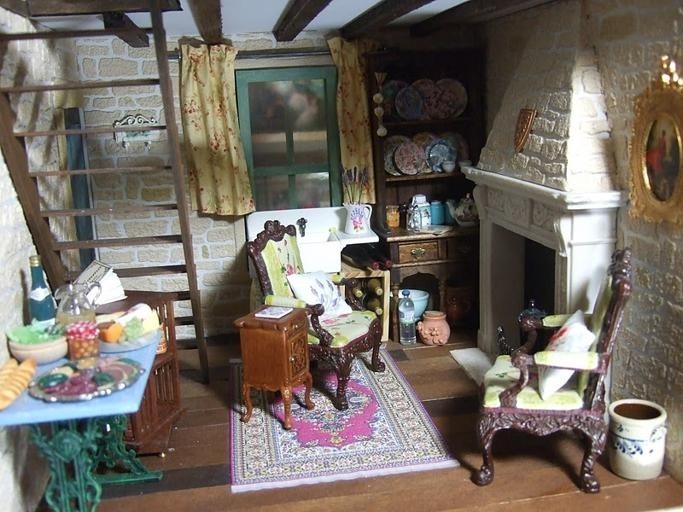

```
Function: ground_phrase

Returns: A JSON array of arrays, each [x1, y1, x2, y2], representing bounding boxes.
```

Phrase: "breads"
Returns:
[[0, 359, 37, 411], [0, 356, 17, 387]]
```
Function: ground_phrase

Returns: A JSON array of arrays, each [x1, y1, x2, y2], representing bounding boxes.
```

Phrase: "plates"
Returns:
[[384, 78, 467, 120], [383, 131, 435, 177], [427, 141, 457, 173]]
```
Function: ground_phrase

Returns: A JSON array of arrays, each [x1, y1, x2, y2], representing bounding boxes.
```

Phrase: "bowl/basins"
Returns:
[[389, 289, 429, 318], [7, 336, 68, 367]]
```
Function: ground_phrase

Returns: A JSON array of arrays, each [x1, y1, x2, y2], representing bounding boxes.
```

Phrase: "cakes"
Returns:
[[98, 321, 123, 343]]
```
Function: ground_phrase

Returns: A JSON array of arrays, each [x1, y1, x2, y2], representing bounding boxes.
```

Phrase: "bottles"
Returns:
[[342, 244, 379, 271], [366, 297, 383, 316], [366, 243, 394, 269], [54, 283, 97, 360], [28, 255, 58, 322], [446, 201, 457, 225], [398, 290, 416, 346], [406, 206, 413, 230], [400, 204, 407, 229], [386, 205, 400, 228], [411, 207, 422, 229], [367, 279, 383, 297], [348, 279, 363, 300], [430, 201, 445, 225]]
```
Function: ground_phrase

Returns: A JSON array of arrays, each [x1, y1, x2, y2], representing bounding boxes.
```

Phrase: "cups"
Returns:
[[458, 160, 471, 168], [442, 161, 455, 172]]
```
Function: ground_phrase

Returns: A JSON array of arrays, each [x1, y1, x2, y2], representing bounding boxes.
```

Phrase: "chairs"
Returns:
[[247, 219, 385, 412], [472, 248, 633, 493]]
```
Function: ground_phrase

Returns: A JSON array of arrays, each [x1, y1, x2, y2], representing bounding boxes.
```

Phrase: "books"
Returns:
[[413, 224, 453, 235], [254, 306, 294, 319]]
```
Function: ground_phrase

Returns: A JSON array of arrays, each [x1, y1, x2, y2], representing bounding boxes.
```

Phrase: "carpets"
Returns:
[[230, 348, 459, 496]]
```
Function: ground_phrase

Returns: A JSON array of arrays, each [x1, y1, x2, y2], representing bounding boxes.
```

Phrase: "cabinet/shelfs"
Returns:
[[237, 307, 314, 431], [370, 66, 483, 342]]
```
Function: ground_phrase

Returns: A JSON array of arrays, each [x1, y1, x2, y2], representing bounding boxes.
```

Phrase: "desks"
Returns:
[[0, 323, 163, 512]]
